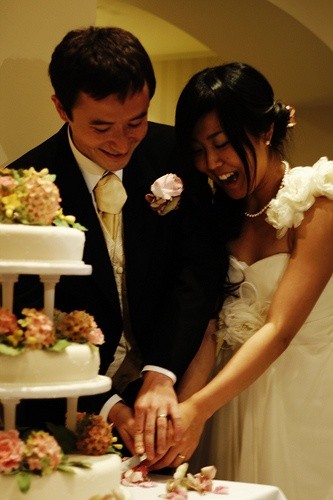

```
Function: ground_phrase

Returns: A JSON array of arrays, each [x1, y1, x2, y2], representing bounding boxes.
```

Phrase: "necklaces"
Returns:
[[242, 160, 289, 217]]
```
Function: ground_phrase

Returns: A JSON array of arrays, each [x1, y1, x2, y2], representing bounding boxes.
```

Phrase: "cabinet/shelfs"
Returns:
[[0, 261, 125, 500]]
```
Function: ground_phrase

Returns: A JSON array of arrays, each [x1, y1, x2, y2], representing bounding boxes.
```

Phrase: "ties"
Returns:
[[94, 175, 127, 243]]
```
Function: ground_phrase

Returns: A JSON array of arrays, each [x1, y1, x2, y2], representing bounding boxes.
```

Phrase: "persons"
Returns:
[[0, 27, 217, 475], [144, 63, 333, 500]]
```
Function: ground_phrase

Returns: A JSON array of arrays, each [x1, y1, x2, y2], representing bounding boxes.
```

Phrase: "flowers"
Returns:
[[145, 173, 183, 216], [0, 167, 88, 232], [0, 308, 105, 356], [122, 457, 227, 500], [0, 412, 124, 495]]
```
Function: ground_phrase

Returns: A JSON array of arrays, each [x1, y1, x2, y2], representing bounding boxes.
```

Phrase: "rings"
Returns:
[[177, 454, 185, 459], [155, 415, 168, 418]]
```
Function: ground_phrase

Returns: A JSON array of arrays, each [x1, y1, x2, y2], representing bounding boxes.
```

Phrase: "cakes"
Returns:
[[0, 165, 123, 500]]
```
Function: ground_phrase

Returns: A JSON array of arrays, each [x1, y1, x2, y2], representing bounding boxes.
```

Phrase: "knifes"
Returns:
[[125, 453, 145, 473]]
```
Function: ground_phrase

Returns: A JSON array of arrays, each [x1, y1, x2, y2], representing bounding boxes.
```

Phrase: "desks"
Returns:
[[122, 473, 287, 500]]
